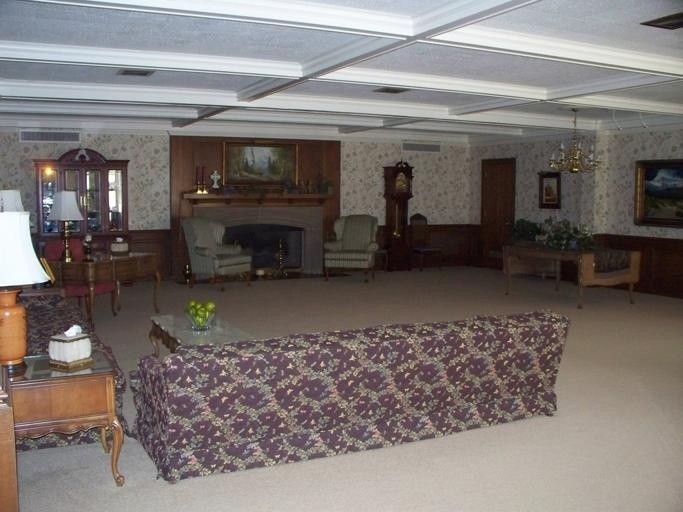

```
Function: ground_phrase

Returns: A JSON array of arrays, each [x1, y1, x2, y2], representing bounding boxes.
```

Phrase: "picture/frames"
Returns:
[[634, 159, 683, 228], [222, 140, 299, 186], [537, 171, 562, 210]]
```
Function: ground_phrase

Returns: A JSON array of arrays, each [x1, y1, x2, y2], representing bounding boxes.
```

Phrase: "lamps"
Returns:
[[548, 107, 604, 176], [0, 210, 51, 367], [45, 190, 85, 264]]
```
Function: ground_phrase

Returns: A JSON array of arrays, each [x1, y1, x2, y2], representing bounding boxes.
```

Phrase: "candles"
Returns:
[[197, 167, 207, 184]]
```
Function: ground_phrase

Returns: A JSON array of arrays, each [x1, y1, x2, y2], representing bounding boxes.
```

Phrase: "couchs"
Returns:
[[130, 310, 570, 485], [6, 288, 130, 452]]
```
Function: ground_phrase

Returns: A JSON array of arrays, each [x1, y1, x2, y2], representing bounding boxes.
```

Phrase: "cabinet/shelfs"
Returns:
[[5, 363, 125, 489], [32, 147, 130, 239]]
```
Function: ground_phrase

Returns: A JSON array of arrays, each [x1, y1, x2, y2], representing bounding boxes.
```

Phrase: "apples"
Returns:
[[186, 299, 216, 326]]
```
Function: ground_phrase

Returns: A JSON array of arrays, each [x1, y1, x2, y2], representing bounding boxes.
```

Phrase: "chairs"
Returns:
[[323, 215, 380, 283], [181, 217, 255, 292], [404, 214, 445, 272]]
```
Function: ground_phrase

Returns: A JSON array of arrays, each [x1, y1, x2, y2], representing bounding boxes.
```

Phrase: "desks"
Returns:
[[501, 242, 641, 310], [55, 253, 163, 325]]
